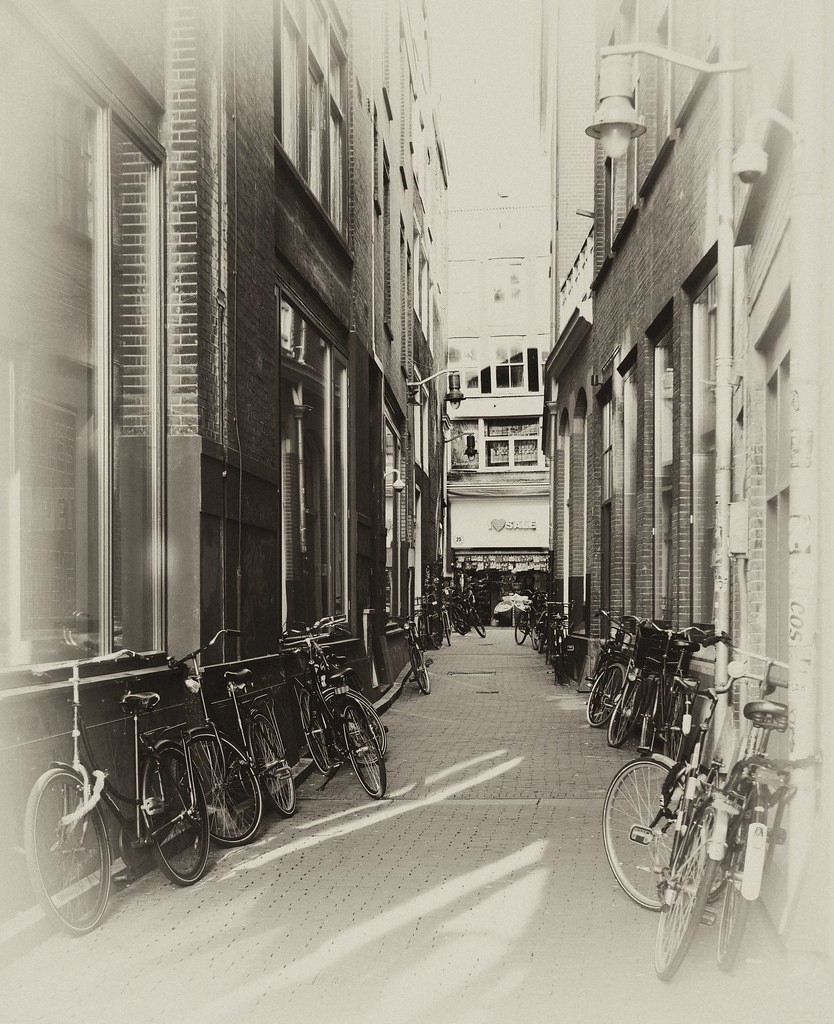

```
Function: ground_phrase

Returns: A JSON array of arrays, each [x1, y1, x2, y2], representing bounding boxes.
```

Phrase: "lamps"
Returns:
[[582, 41, 757, 161], [443, 431, 480, 462], [408, 370, 469, 410], [590, 374, 603, 386]]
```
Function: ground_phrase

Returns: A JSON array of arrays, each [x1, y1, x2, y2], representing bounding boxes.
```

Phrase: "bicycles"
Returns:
[[601, 658, 795, 980], [699, 639, 796, 974], [585, 609, 728, 765], [57, 610, 126, 657], [381, 609, 437, 695], [275, 613, 398, 800], [418, 577, 488, 642], [515, 587, 577, 688], [145, 626, 297, 848], [22, 648, 211, 938]]
